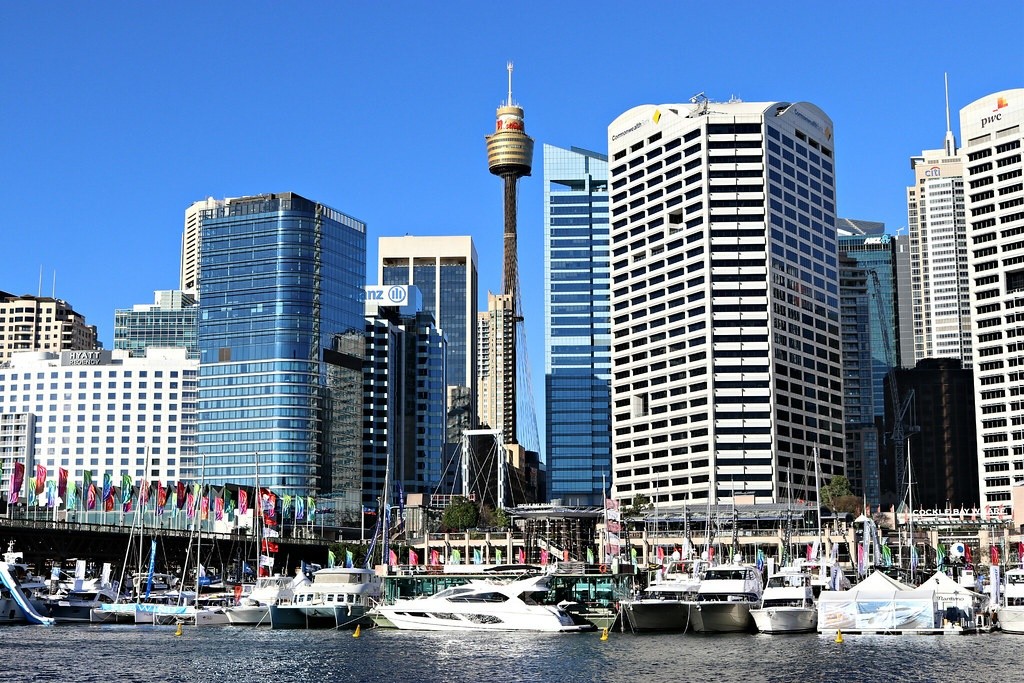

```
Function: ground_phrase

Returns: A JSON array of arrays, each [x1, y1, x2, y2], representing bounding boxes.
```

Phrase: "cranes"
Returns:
[[872, 270, 921, 514]]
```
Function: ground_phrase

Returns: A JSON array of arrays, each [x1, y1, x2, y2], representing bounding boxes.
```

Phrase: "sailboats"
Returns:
[[1, 454, 1024, 636]]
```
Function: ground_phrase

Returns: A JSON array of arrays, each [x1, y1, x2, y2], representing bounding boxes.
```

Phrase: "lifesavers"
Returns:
[[598, 564, 608, 575]]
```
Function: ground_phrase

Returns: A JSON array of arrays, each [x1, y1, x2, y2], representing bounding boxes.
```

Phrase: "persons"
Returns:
[[466, 529, 472, 540], [508, 529, 514, 539]]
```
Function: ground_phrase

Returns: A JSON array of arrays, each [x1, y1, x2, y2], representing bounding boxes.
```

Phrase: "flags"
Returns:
[[300, 549, 354, 572], [243, 528, 279, 578], [606, 498, 621, 563], [806, 546, 817, 568], [199, 564, 211, 586], [9, 461, 316, 525], [855, 503, 1024, 575], [51, 567, 60, 580], [629, 542, 788, 574], [101, 563, 111, 588], [387, 548, 594, 567], [74, 560, 86, 581], [364, 507, 377, 515]]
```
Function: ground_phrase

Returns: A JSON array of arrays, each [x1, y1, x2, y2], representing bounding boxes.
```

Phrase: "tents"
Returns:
[[818, 570, 985, 629]]
